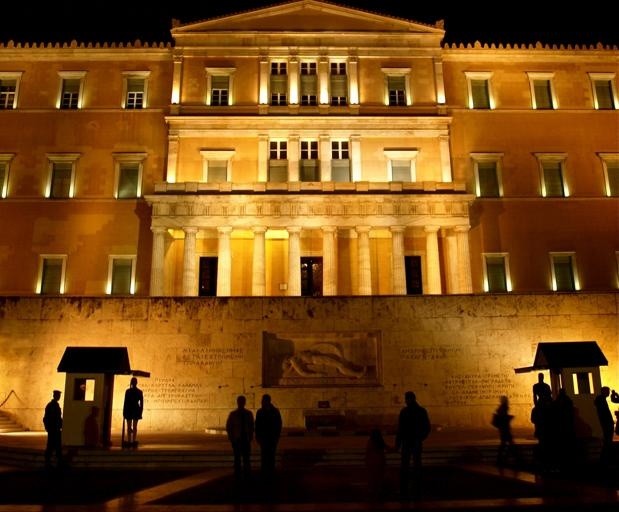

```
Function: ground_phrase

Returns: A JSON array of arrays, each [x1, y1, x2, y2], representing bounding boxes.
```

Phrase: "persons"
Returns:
[[489, 396, 525, 472], [43, 390, 65, 472], [122, 377, 144, 448], [365, 391, 430, 479], [225, 393, 283, 477], [532, 372, 614, 444]]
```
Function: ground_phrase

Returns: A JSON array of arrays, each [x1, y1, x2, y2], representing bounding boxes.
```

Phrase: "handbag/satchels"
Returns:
[[491, 412, 501, 429]]
